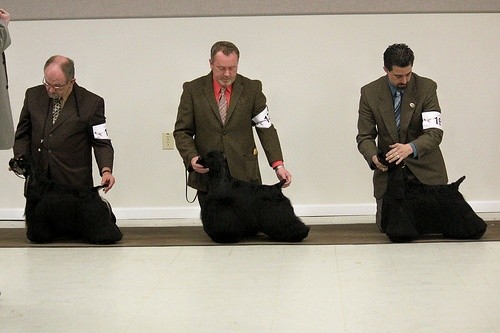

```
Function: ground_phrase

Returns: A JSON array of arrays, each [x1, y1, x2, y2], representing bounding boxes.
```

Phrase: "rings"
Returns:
[[397, 154, 399, 158]]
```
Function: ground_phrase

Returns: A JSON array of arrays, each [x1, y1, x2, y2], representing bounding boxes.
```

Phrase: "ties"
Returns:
[[52, 97, 62, 125], [394, 91, 401, 140], [218, 87, 228, 125]]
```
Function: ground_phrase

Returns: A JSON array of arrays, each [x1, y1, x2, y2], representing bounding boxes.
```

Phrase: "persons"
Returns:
[[173, 41, 291, 207], [8, 55, 115, 197], [0, 8, 14, 151], [356, 43, 448, 232]]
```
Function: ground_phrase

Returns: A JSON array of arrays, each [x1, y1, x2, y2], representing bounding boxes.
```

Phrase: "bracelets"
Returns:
[[102, 169, 111, 175], [274, 164, 284, 171]]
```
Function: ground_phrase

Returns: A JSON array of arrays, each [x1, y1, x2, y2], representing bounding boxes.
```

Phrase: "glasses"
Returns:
[[41, 76, 69, 90]]
